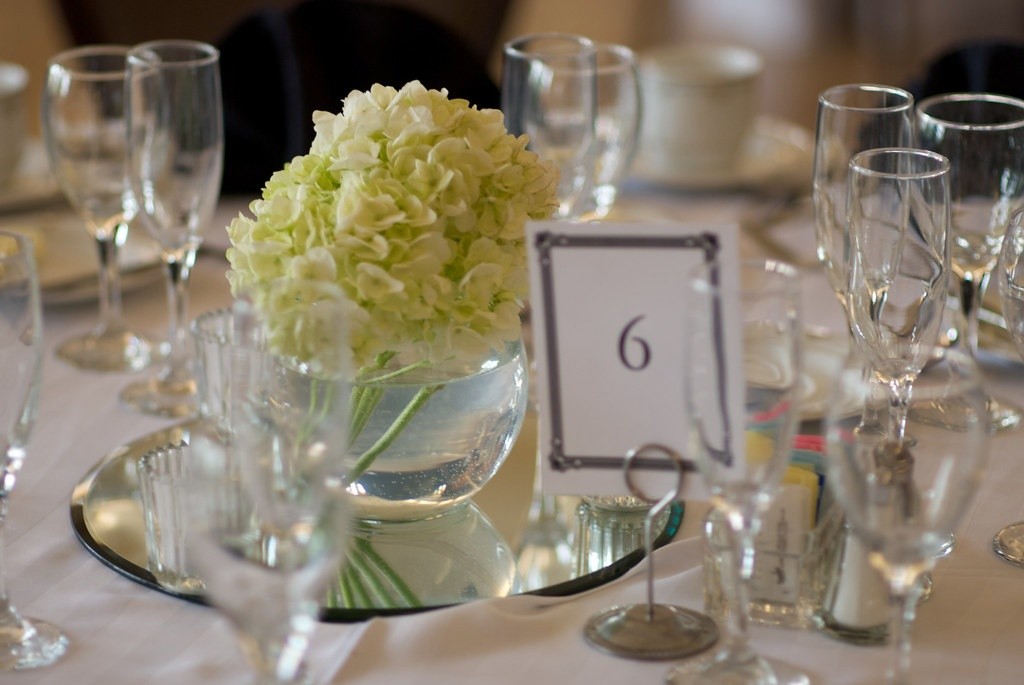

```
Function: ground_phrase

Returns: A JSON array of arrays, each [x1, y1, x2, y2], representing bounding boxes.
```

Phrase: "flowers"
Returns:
[[223, 81, 559, 491]]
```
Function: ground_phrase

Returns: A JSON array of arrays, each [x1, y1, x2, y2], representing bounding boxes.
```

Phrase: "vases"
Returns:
[[254, 501, 518, 610], [274, 331, 528, 525]]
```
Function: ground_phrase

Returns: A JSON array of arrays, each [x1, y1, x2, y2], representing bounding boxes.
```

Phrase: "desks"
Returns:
[[1, 185, 1024, 685]]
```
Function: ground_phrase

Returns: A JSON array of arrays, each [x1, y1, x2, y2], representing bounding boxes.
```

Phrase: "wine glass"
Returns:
[[119, 43, 225, 418], [669, 81, 1024, 685], [0, 231, 70, 669], [185, 303, 356, 685], [41, 44, 168, 376]]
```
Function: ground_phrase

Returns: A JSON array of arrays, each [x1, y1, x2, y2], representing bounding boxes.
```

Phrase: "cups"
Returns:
[[636, 42, 763, 188], [501, 30, 598, 217], [526, 42, 639, 225]]
